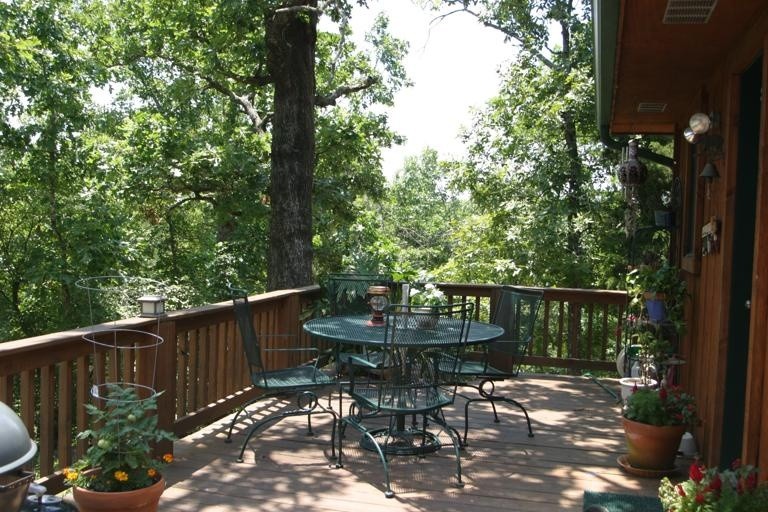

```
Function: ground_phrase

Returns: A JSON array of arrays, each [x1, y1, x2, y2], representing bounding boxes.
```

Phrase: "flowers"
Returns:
[[62, 384, 176, 490], [621, 380, 704, 432], [658, 458, 767, 511]]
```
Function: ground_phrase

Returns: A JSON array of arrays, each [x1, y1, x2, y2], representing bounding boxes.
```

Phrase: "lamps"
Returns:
[[682, 108, 721, 201]]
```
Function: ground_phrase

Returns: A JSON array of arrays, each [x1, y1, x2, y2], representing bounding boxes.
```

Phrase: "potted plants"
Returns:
[[618, 263, 689, 406]]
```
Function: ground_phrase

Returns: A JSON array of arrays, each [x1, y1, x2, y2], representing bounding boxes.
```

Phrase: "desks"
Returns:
[[302, 312, 505, 456]]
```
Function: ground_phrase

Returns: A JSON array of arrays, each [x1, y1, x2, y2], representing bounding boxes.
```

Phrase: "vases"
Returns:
[[72, 462, 166, 512], [616, 416, 684, 479]]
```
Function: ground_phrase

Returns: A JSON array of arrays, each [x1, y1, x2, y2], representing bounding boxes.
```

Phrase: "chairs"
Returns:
[[336, 302, 474, 497], [227, 283, 338, 464], [326, 278, 418, 410], [432, 285, 545, 447]]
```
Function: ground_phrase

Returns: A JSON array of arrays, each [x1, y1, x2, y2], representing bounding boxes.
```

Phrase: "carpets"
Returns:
[[582, 488, 663, 512]]
